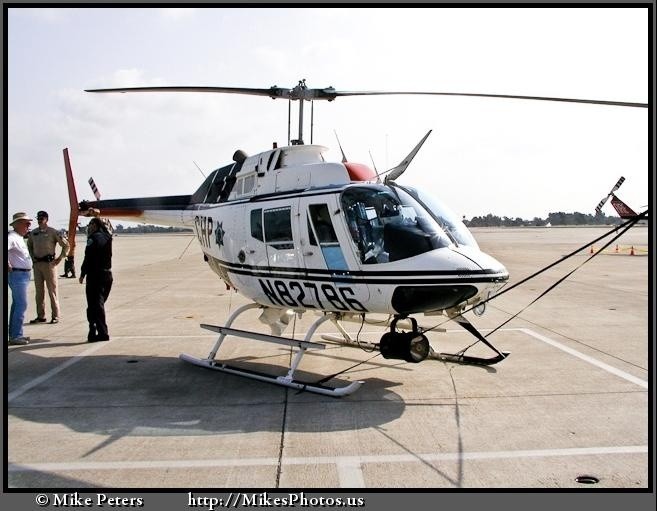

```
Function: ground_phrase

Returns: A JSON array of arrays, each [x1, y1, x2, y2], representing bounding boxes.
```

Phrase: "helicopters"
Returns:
[[62, 77, 647, 396], [595, 176, 648, 220]]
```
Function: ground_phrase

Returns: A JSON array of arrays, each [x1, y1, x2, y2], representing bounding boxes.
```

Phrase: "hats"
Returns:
[[9, 213, 34, 225], [37, 211, 48, 218]]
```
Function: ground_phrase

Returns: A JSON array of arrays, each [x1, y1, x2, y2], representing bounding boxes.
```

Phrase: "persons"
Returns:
[[59, 237, 76, 278], [79, 208, 112, 341], [27, 211, 70, 324], [8, 212, 35, 345]]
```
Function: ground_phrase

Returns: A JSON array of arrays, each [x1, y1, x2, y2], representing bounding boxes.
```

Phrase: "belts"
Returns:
[[12, 268, 31, 272]]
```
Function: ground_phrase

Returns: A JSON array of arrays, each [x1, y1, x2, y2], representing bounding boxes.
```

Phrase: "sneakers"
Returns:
[[30, 317, 46, 323], [95, 335, 109, 341], [51, 318, 59, 323], [88, 326, 96, 342], [10, 337, 30, 345]]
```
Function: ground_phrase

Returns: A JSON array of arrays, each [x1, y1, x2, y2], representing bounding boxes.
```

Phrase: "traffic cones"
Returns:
[[630, 245, 634, 255], [615, 244, 619, 253], [590, 247, 593, 254]]
